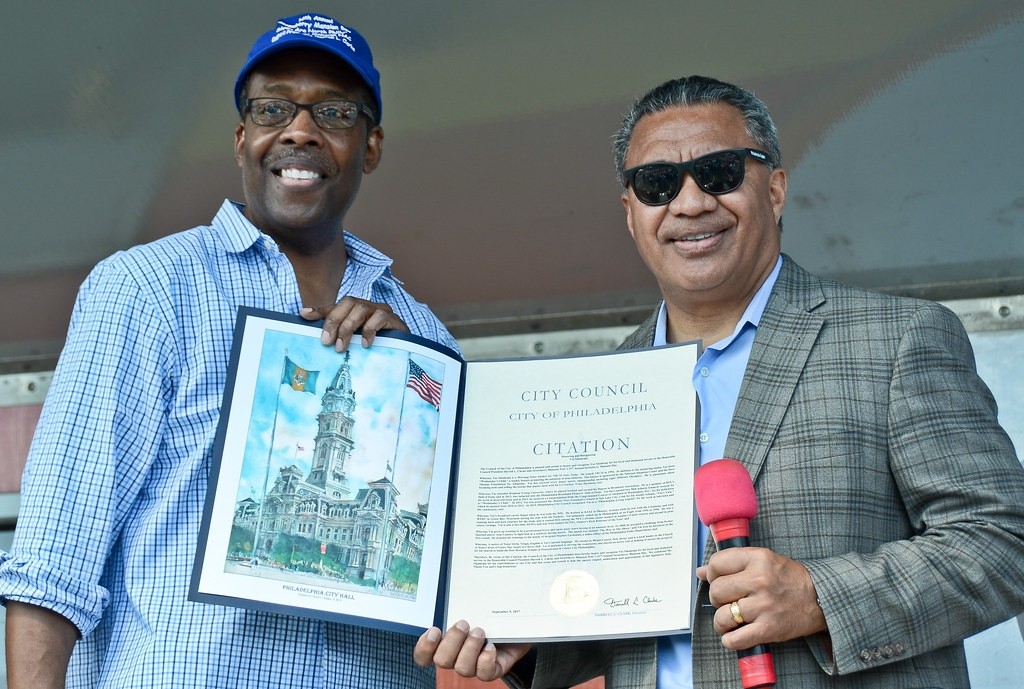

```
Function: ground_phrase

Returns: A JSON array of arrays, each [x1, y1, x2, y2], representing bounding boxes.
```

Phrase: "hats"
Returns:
[[233, 13, 382, 126]]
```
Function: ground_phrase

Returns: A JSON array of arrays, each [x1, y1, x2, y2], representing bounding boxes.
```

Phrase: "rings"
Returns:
[[730, 600, 744, 625]]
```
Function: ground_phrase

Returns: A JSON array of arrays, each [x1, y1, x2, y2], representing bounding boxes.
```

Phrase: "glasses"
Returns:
[[621, 146, 774, 207], [242, 96, 376, 131]]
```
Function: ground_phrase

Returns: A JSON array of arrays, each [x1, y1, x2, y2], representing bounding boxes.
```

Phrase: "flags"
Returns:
[[407, 358, 441, 413], [281, 354, 321, 395]]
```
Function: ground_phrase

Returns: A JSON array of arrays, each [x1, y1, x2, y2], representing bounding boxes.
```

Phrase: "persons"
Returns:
[[414, 77, 1023, 687], [1, 14, 469, 687]]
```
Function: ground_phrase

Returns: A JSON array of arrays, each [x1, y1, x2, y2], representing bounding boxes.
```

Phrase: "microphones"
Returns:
[[694, 459, 776, 689]]
